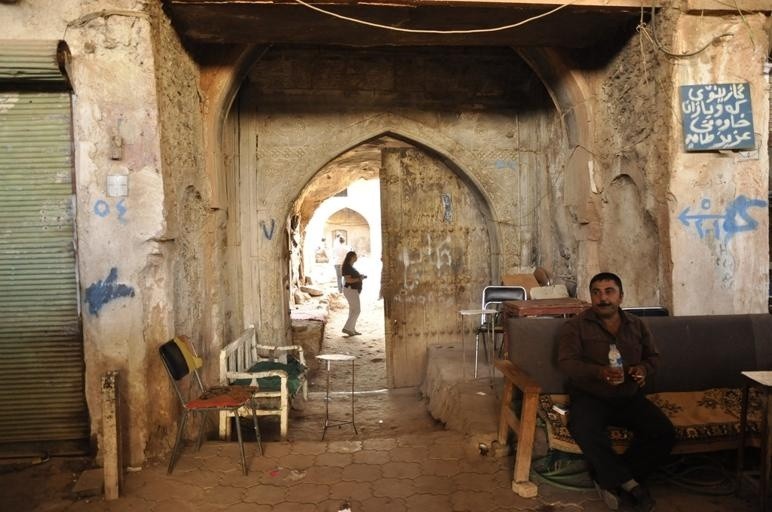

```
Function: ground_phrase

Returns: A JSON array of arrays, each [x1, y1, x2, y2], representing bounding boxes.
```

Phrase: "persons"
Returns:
[[332, 236, 352, 293], [342, 251, 367, 336], [317, 238, 328, 251], [556, 271, 676, 512], [334, 233, 342, 246]]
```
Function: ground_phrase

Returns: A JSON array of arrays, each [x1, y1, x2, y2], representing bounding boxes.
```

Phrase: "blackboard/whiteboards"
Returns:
[[678, 82, 756, 152]]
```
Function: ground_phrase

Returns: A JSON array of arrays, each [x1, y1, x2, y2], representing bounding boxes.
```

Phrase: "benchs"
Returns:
[[500, 314, 772, 499], [220, 324, 308, 441]]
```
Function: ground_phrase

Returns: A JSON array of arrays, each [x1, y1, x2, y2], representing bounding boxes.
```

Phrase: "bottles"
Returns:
[[608, 344, 624, 384]]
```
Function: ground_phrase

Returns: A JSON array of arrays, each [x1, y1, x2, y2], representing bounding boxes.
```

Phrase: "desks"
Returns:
[[733, 369, 772, 510], [502, 296, 592, 361], [314, 352, 360, 441], [456, 308, 498, 384]]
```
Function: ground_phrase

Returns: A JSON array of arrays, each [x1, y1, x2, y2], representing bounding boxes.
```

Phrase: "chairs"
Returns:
[[156, 335, 263, 475], [471, 285, 570, 379]]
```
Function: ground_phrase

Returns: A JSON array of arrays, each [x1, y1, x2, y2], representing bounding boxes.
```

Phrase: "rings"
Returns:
[[606, 377, 610, 380]]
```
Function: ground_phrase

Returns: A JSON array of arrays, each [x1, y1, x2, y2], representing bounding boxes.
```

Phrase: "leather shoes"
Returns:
[[626, 482, 656, 512], [592, 479, 623, 510]]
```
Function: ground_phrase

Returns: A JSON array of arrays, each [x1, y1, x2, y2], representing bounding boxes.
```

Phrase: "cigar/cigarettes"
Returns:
[[631, 375, 643, 378]]
